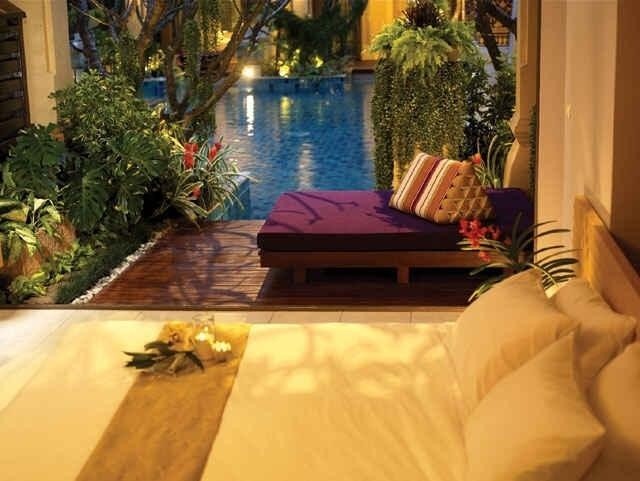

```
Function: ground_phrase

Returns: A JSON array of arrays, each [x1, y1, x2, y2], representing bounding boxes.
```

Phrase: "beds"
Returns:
[[0, 197, 640, 481], [257, 188, 533, 284]]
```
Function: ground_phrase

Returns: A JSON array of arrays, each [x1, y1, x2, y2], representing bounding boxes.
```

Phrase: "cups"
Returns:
[[191, 313, 216, 359]]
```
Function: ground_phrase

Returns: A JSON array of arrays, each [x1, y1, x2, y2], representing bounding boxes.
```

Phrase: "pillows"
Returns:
[[586, 342, 640, 481], [388, 149, 498, 223], [545, 277, 637, 393], [442, 268, 581, 414], [463, 333, 606, 481]]
